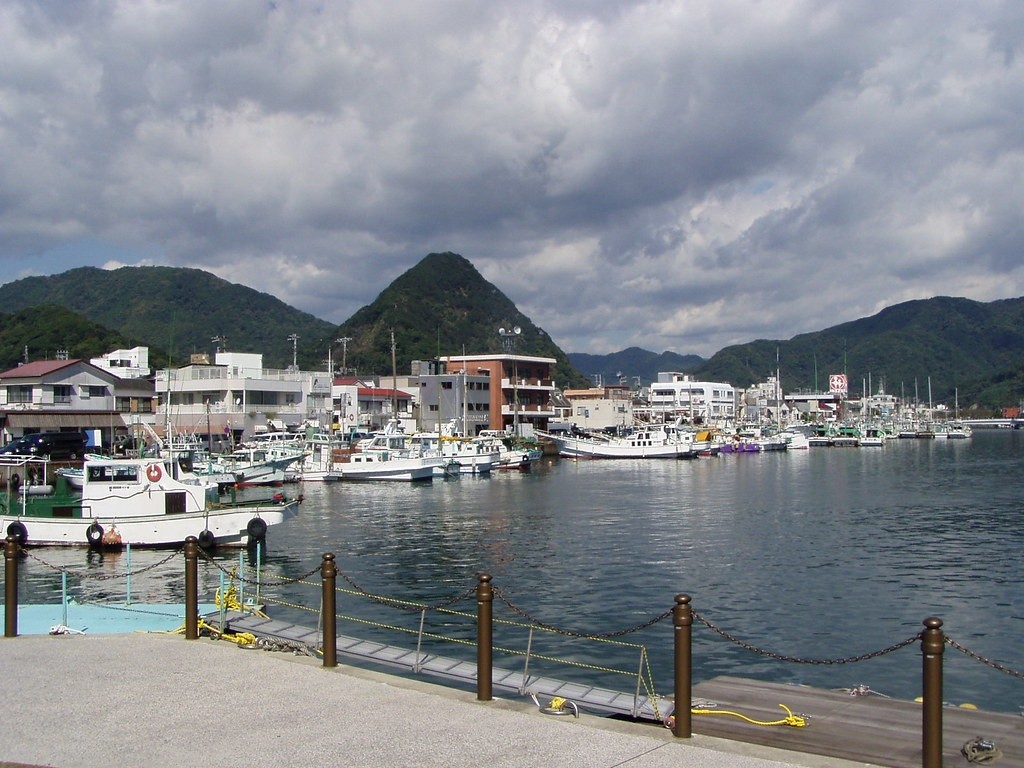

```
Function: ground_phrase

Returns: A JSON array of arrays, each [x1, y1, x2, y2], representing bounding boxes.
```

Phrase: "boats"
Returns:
[[532, 385, 790, 459], [0, 458, 299, 549], [50, 415, 542, 483]]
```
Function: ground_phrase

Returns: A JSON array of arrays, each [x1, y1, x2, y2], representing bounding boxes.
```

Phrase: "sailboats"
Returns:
[[770, 338, 973, 450]]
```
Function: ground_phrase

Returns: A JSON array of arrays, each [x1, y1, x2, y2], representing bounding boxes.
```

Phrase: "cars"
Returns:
[[114, 435, 127, 444], [116, 438, 146, 454], [454, 432, 472, 439], [0, 439, 21, 456]]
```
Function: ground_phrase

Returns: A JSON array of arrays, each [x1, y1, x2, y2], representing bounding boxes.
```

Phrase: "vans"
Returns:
[[189, 432, 231, 455], [478, 429, 506, 438], [312, 433, 340, 449], [16, 432, 86, 459]]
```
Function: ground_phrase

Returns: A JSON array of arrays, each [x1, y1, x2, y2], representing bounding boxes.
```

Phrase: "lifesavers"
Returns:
[[522, 455, 528, 462], [147, 464, 162, 482], [6, 522, 28, 543], [85, 524, 104, 544], [12, 474, 20, 484]]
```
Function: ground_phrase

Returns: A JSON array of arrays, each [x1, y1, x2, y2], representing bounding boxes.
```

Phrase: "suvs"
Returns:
[[605, 426, 631, 436]]
